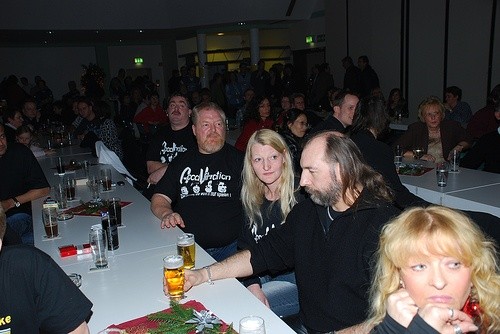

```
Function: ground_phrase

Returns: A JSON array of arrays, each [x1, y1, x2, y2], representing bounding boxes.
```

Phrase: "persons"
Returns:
[[392, 84, 500, 174], [344, 97, 420, 207], [358, 55, 380, 89], [277, 109, 309, 179], [369, 206, 500, 334], [0, 206, 93, 334], [127, 95, 198, 187], [0, 58, 408, 151], [164, 131, 400, 334], [0, 126, 50, 218], [150, 102, 245, 263], [237, 129, 305, 316]]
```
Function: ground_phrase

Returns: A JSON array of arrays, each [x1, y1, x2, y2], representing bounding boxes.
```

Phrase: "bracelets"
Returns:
[[456, 144, 464, 152], [247, 284, 259, 289], [202, 265, 214, 285]]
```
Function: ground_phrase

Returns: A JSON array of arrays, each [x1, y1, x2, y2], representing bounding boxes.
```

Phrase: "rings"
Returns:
[[431, 155, 432, 158], [449, 308, 454, 318], [454, 324, 461, 334]]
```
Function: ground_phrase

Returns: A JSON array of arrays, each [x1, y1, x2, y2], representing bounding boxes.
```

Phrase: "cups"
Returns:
[[436, 161, 449, 187], [177, 233, 195, 271], [42, 207, 58, 238], [450, 150, 461, 172], [87, 164, 112, 202], [53, 183, 68, 210], [63, 176, 76, 201], [89, 229, 109, 269], [163, 255, 185, 301], [101, 215, 119, 251], [238, 315, 266, 334], [106, 197, 121, 227]]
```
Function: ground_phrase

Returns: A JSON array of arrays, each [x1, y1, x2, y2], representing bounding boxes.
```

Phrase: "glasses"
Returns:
[[295, 121, 309, 128]]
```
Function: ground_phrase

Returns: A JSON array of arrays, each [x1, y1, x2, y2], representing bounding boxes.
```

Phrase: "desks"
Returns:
[[31, 144, 298, 334], [394, 154, 500, 219]]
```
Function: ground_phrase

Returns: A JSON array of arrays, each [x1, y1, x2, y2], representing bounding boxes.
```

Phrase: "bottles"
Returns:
[[42, 118, 72, 150]]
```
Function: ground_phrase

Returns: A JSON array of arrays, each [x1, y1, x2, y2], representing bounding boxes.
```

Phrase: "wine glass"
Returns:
[[413, 147, 425, 160], [393, 152, 402, 173]]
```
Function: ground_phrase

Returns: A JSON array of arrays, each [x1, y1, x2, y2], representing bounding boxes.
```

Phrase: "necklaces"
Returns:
[[327, 201, 335, 220], [428, 125, 440, 133]]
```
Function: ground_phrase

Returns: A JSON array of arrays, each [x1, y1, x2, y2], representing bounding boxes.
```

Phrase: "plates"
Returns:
[[398, 167, 434, 177]]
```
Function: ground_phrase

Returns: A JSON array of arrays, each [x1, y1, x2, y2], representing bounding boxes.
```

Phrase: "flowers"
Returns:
[[145, 300, 229, 334]]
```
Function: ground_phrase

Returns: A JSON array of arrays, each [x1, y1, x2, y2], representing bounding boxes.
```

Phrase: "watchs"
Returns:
[[10, 197, 21, 207], [449, 105, 453, 112]]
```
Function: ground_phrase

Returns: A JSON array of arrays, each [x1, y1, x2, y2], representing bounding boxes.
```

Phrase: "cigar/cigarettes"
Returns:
[[147, 182, 150, 189]]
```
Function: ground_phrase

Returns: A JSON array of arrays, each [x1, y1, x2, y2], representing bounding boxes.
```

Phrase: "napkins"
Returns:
[[95, 141, 138, 181]]
